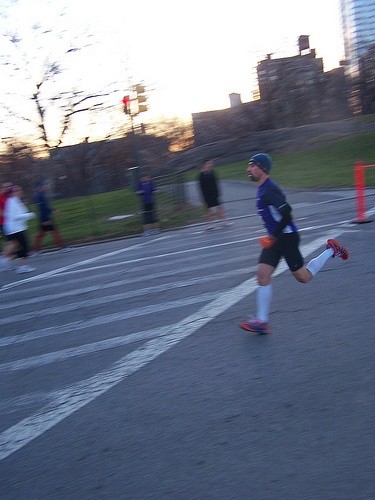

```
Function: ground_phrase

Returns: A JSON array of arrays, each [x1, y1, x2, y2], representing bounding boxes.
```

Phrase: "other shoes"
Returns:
[[16, 264, 37, 274]]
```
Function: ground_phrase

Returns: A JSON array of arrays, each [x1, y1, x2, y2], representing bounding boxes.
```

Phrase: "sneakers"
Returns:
[[325, 239, 349, 262], [239, 318, 272, 333]]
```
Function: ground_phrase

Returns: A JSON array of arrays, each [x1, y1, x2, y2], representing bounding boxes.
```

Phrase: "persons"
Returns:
[[237, 153, 350, 335], [0, 185, 38, 274], [132, 168, 161, 238], [198, 158, 234, 231], [34, 183, 68, 251], [0, 182, 19, 258]]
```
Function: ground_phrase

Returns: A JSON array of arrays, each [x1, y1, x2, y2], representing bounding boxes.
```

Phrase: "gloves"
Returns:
[[259, 235, 276, 249]]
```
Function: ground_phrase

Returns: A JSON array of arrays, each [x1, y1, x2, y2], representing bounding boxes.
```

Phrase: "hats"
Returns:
[[249, 152, 272, 173]]
